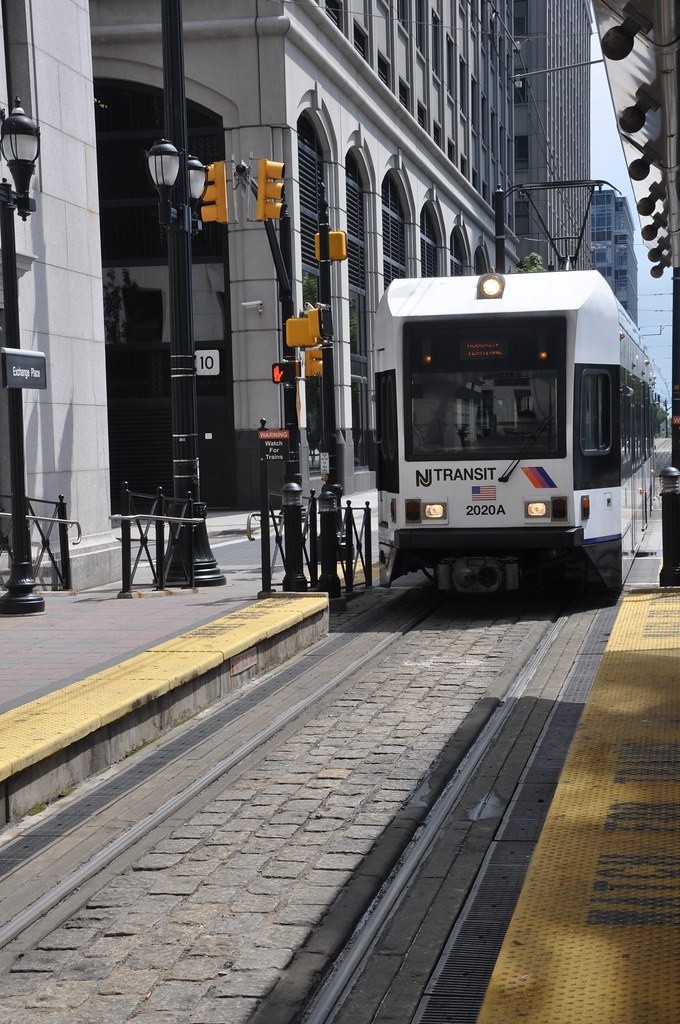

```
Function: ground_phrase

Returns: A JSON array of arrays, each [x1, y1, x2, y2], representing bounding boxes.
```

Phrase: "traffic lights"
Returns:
[[270, 361, 297, 385], [257, 156, 288, 223], [198, 163, 230, 223], [302, 348, 323, 377], [307, 307, 325, 339]]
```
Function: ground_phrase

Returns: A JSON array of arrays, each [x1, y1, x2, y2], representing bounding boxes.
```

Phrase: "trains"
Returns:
[[345, 181, 661, 606]]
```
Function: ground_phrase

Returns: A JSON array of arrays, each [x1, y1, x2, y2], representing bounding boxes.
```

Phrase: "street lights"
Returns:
[[143, 137, 228, 588], [0, 98, 52, 616]]
